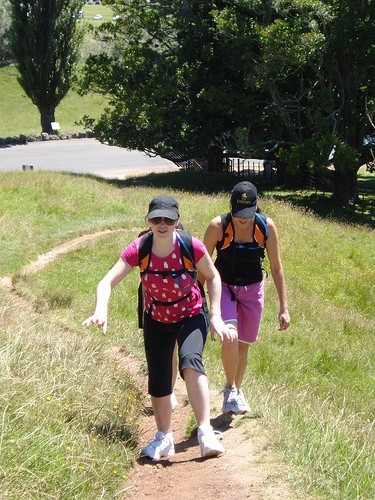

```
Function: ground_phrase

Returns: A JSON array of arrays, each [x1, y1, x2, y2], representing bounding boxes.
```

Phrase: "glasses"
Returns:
[[149, 216, 176, 226]]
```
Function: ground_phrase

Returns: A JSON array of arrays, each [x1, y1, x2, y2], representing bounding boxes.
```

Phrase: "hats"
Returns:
[[148, 195, 180, 220], [230, 180, 256, 219]]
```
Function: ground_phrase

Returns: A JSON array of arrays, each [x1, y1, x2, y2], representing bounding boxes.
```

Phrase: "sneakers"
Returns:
[[196, 425, 224, 457], [141, 429, 175, 462], [232, 392, 250, 415], [219, 387, 236, 415]]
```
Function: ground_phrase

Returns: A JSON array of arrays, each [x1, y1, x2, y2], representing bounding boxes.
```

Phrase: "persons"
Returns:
[[196, 181, 291, 414], [81, 195, 234, 460]]
